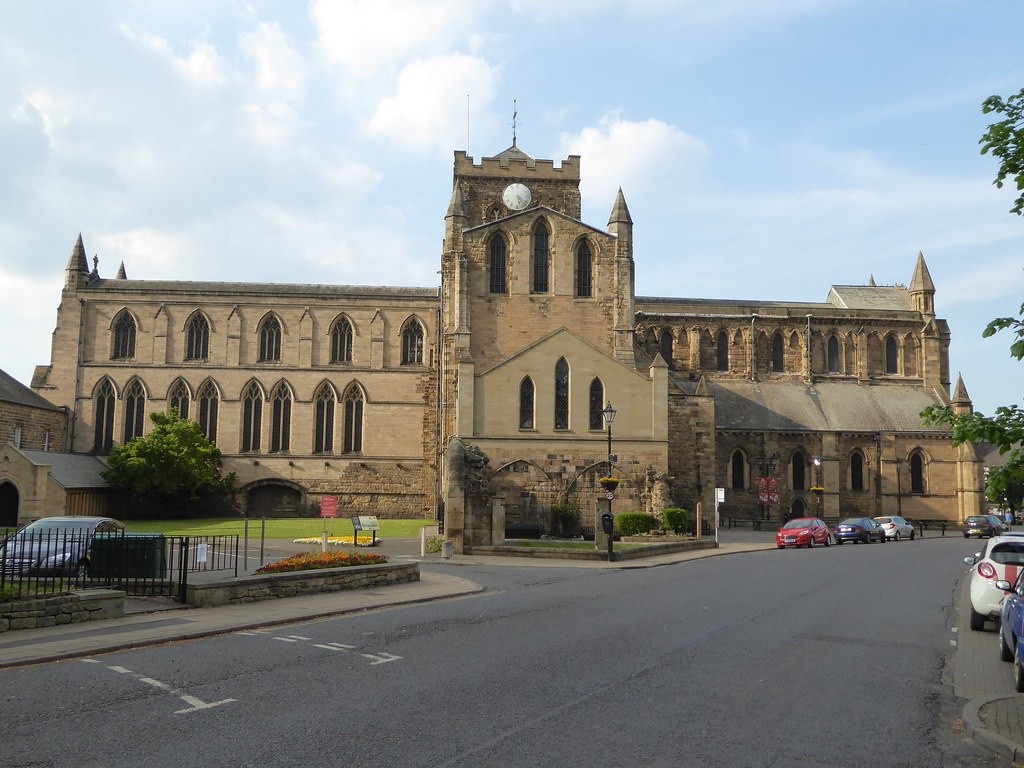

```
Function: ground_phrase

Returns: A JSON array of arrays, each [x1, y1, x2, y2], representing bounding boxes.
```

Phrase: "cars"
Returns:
[[996, 567, 1024, 694], [833, 516, 886, 545], [962, 515, 1010, 539], [0, 516, 130, 579], [964, 536, 1024, 631], [776, 517, 832, 549], [873, 515, 915, 541]]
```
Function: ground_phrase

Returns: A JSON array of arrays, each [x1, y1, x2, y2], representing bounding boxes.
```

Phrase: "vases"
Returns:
[[601, 481, 618, 491], [812, 489, 824, 495]]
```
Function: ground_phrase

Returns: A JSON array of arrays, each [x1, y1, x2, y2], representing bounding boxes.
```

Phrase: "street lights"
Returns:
[[814, 458, 823, 516], [601, 399, 618, 512]]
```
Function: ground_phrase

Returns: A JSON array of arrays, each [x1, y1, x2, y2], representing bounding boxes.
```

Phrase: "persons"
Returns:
[[784, 513, 794, 527], [1005, 510, 1013, 532]]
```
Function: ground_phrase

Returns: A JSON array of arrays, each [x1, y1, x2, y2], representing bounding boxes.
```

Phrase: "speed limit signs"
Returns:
[[605, 492, 614, 500]]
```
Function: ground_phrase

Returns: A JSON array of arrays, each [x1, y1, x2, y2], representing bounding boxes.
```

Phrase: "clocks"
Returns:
[[502, 182, 532, 211]]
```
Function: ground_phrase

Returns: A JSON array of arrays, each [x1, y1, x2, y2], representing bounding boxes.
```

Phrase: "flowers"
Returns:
[[809, 486, 824, 491], [598, 476, 619, 482]]
